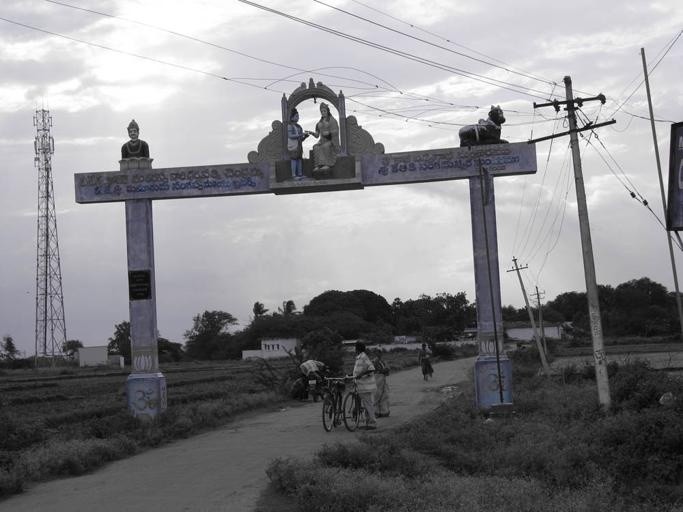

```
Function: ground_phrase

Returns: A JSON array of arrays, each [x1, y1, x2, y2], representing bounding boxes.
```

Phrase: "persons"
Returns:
[[121, 117, 148, 159], [290, 376, 309, 402], [287, 109, 310, 182], [417, 342, 435, 381], [304, 101, 341, 171], [298, 356, 326, 386], [352, 340, 379, 430], [370, 346, 392, 418]]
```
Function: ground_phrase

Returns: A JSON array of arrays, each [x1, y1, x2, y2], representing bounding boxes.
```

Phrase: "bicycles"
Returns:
[[341, 375, 367, 432], [319, 373, 345, 433]]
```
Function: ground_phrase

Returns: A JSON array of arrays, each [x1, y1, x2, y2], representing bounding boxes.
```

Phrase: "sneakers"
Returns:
[[364, 425, 376, 430]]
